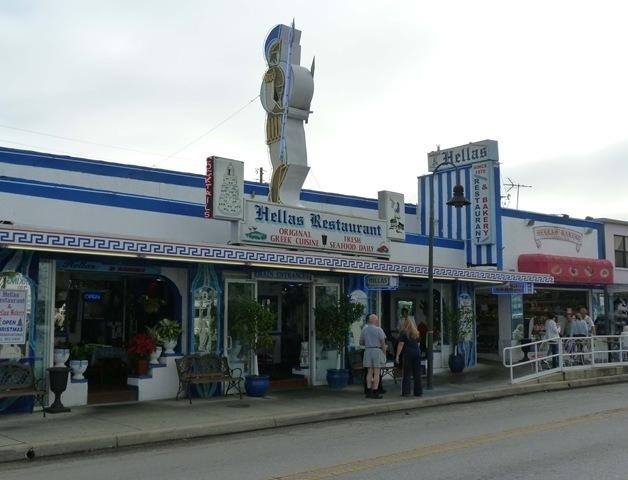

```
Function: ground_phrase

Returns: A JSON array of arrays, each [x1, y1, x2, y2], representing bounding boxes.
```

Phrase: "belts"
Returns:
[[364, 346, 382, 349]]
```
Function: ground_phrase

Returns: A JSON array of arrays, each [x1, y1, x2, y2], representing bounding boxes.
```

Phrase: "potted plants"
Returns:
[[69, 342, 97, 380], [147, 318, 184, 365], [442, 297, 479, 373], [231, 294, 276, 397], [311, 297, 365, 391]]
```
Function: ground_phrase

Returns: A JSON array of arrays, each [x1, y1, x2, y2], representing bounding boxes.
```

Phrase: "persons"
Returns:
[[395, 319, 424, 397], [395, 308, 418, 330], [542, 307, 596, 370]]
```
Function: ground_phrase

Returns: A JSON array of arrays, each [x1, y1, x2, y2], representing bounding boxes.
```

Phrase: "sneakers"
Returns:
[[364, 388, 422, 399]]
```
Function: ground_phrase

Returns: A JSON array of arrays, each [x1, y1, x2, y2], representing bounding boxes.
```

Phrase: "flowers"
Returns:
[[127, 334, 155, 357]]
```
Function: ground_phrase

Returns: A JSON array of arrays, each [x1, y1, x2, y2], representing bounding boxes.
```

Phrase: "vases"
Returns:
[[45, 367, 71, 414], [136, 360, 147, 376], [52, 347, 69, 368]]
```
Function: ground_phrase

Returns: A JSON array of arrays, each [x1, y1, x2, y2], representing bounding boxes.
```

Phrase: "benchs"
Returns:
[[1, 362, 49, 418], [175, 353, 245, 404], [348, 350, 402, 393]]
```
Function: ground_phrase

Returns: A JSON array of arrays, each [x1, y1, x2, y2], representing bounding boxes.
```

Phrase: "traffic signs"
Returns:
[[428, 161, 472, 395]]
